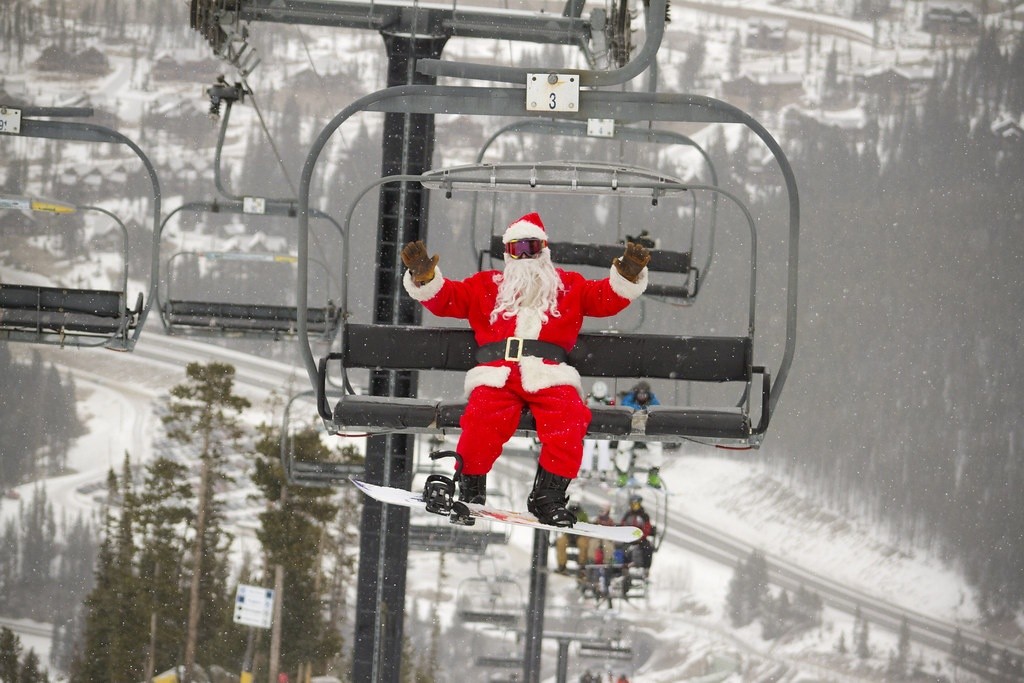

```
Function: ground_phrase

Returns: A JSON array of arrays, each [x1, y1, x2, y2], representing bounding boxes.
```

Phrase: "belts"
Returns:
[[476, 337, 567, 362]]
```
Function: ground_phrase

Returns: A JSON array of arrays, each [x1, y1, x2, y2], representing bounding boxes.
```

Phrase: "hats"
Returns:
[[639, 381, 649, 393], [592, 381, 607, 398], [503, 212, 547, 244]]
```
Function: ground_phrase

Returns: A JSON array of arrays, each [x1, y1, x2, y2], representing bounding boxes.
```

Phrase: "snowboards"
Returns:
[[346, 475, 644, 545]]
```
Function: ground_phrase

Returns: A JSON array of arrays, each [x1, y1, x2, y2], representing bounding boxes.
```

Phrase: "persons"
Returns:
[[401, 214, 650, 529], [556, 500, 588, 571], [587, 501, 615, 566], [587, 382, 663, 489], [580, 670, 629, 683], [620, 496, 652, 583]]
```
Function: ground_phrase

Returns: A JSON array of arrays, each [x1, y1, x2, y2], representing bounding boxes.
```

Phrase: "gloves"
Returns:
[[613, 242, 650, 282], [401, 241, 439, 287]]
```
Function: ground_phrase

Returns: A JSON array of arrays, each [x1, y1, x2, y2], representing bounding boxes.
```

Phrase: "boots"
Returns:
[[648, 467, 660, 485], [457, 471, 486, 505], [527, 462, 577, 527], [616, 466, 630, 487]]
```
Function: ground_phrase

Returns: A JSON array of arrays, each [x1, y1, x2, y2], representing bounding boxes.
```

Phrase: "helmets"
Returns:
[[629, 494, 642, 503]]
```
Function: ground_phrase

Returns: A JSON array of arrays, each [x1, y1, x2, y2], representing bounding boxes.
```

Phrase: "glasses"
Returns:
[[506, 238, 545, 260]]
